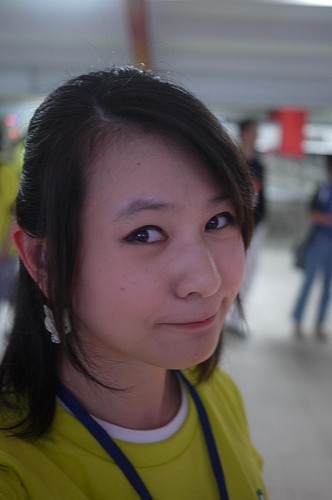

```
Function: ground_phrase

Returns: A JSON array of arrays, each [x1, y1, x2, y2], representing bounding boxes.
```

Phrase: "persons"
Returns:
[[237, 119, 267, 222], [0, 66, 269, 500], [290, 155, 332, 342]]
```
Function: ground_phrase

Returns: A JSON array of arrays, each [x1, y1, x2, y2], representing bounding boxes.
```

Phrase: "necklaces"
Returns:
[[57, 370, 228, 500]]
